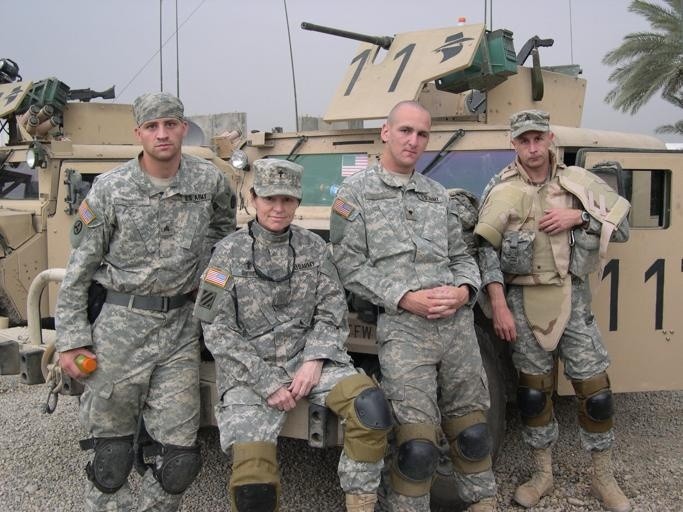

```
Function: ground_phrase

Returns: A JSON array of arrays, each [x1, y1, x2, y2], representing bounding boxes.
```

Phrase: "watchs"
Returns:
[[581, 206, 591, 229]]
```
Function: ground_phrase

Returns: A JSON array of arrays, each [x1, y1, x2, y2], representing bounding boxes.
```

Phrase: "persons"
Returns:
[[192, 158, 394, 512], [329, 101, 499, 512], [472, 109, 632, 512], [55, 92, 237, 511]]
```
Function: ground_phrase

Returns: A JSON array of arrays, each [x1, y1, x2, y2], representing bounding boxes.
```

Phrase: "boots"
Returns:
[[512, 446, 560, 509], [342, 492, 379, 512], [587, 444, 632, 512], [468, 494, 502, 512]]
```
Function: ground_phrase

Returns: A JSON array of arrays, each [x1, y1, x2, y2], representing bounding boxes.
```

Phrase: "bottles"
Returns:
[[74, 354, 96, 375]]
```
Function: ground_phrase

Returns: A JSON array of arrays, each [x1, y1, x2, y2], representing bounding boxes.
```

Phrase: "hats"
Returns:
[[508, 109, 551, 140], [252, 158, 305, 202]]
